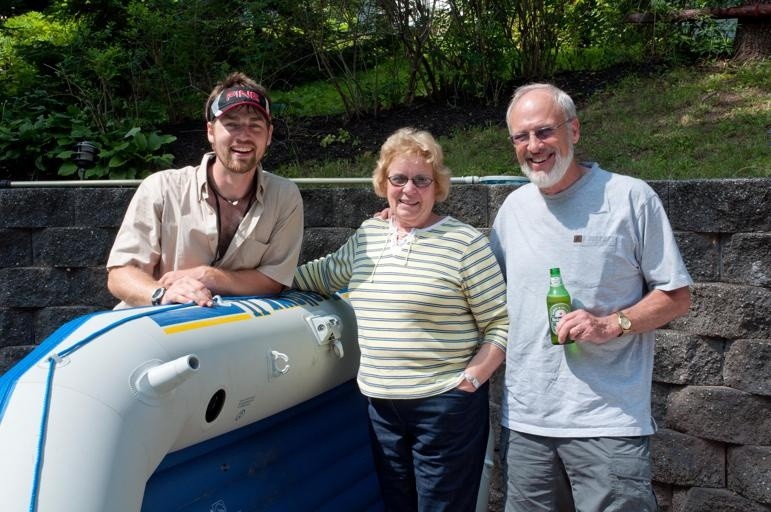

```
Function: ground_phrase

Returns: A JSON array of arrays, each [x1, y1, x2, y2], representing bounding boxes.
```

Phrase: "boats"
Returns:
[[0, 286, 381, 511]]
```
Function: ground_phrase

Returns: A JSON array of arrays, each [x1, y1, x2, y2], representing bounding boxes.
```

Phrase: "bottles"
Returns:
[[546, 268, 575, 345]]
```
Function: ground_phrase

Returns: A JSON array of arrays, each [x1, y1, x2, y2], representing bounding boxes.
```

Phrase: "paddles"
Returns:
[[0, 175, 531, 187]]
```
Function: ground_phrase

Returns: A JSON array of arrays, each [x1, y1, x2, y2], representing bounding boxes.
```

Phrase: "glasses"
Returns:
[[384, 174, 437, 189], [505, 118, 573, 147]]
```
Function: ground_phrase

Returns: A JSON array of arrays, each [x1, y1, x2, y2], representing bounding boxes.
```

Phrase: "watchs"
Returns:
[[612, 309, 634, 339], [150, 285, 166, 306], [462, 371, 481, 393]]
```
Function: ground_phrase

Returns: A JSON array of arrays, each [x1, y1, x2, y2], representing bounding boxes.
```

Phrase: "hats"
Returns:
[[207, 84, 272, 123]]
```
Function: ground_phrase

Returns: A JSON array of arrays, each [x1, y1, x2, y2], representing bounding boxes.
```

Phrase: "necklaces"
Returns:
[[206, 177, 254, 205]]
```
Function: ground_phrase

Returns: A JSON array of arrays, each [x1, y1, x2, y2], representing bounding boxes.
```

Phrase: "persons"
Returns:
[[370, 81, 695, 512], [105, 69, 305, 313], [287, 128, 509, 512]]
